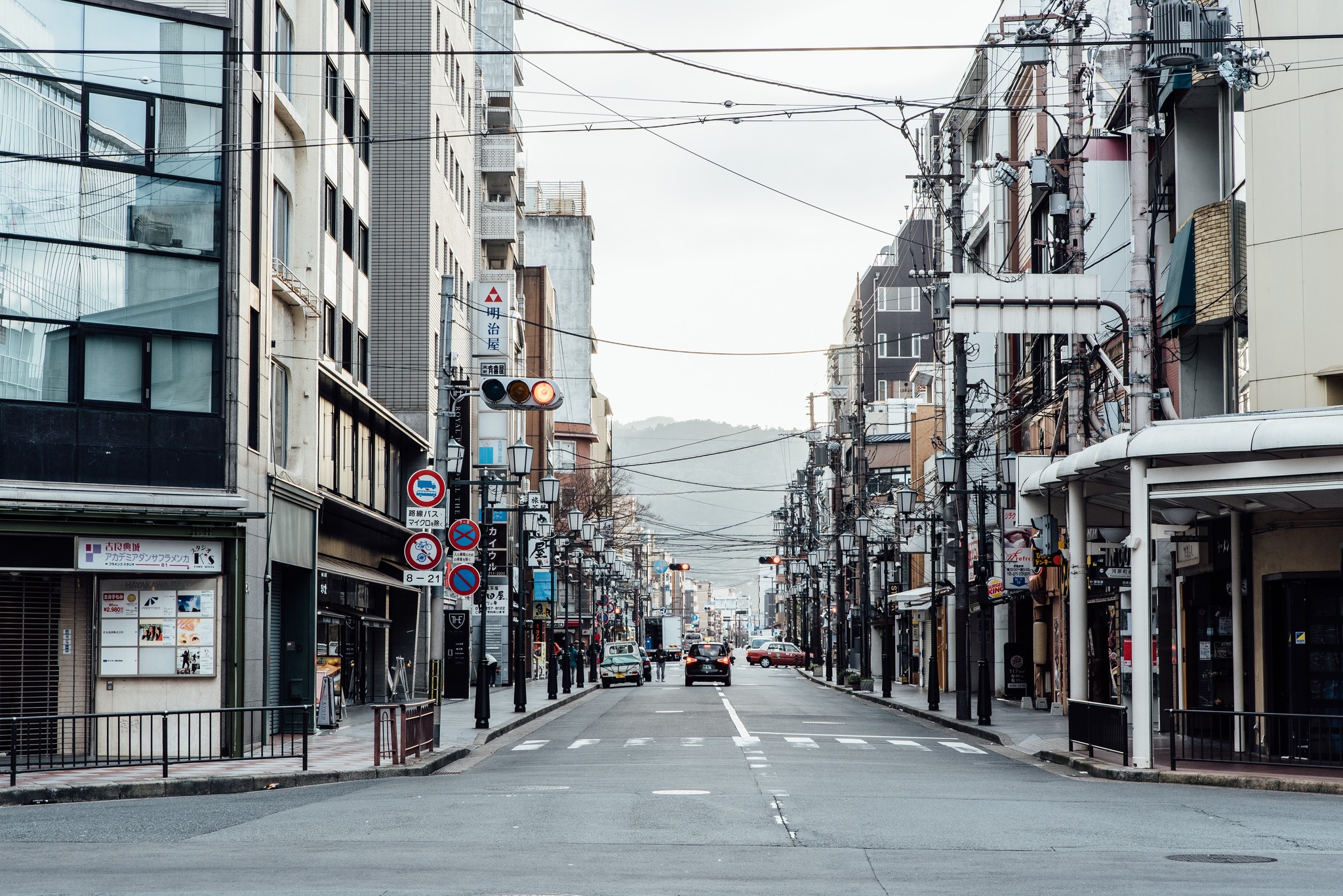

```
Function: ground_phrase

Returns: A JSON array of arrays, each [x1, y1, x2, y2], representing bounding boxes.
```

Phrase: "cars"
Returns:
[[746, 642, 825, 668], [638, 647, 652, 682]]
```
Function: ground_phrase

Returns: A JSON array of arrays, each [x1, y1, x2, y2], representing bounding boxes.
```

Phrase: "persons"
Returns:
[[726, 641, 735, 665], [554, 642, 560, 676], [723, 638, 729, 656], [655, 643, 668, 682], [589, 639, 601, 654], [777, 633, 783, 642], [568, 643, 578, 685], [597, 639, 602, 658]]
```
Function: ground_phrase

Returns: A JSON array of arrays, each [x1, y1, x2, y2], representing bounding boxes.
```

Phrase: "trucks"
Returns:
[[643, 616, 683, 662], [599, 640, 645, 688], [748, 636, 775, 665]]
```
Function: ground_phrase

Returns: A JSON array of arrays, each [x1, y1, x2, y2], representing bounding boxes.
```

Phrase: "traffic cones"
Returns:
[[317, 677, 337, 729], [328, 676, 340, 728]]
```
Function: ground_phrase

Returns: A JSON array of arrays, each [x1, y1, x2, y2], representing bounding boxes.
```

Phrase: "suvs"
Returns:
[[683, 639, 735, 687]]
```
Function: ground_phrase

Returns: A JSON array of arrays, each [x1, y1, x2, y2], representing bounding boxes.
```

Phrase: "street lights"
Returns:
[[598, 565, 633, 641], [775, 589, 784, 630], [589, 561, 627, 643], [538, 518, 595, 694], [555, 532, 605, 688], [789, 484, 918, 697], [583, 555, 623, 679], [486, 469, 560, 713], [446, 435, 534, 726], [627, 578, 654, 649], [522, 505, 584, 699], [936, 449, 1018, 725], [572, 546, 617, 683]]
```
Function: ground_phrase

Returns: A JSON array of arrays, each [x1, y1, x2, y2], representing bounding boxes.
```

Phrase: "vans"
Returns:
[[683, 628, 703, 654]]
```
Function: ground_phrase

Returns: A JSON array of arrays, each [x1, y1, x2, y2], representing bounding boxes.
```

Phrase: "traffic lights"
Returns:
[[479, 377, 562, 407], [669, 563, 690, 570], [691, 604, 758, 643], [759, 556, 781, 564]]
